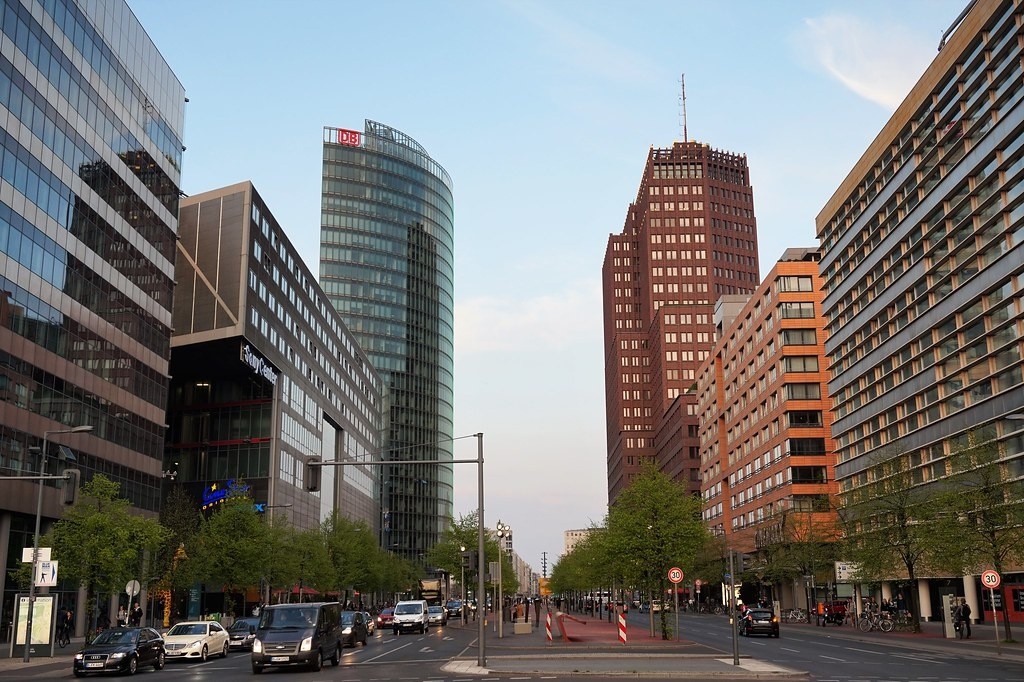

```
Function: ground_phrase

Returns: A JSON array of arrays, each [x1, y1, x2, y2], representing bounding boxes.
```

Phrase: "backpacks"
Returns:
[[960, 604, 971, 617]]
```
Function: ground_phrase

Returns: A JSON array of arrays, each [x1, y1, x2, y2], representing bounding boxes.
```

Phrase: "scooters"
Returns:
[[822, 607, 844, 627]]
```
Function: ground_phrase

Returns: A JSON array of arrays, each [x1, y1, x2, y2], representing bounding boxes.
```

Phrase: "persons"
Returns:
[[951, 598, 971, 639], [844, 594, 911, 632], [132, 603, 143, 626], [349, 601, 354, 611], [359, 601, 363, 611], [115, 604, 125, 627], [97, 612, 111, 630], [57, 606, 75, 644], [384, 601, 388, 608]]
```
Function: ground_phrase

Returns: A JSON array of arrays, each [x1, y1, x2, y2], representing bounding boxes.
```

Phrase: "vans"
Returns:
[[822, 599, 850, 618], [391, 599, 430, 635], [249, 601, 343, 675]]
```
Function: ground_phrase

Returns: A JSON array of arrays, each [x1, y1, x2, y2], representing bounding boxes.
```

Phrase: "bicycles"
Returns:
[[55, 621, 71, 648], [681, 598, 730, 616], [859, 605, 897, 633], [787, 607, 809, 624]]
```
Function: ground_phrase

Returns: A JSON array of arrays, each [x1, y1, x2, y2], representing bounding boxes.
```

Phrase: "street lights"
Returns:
[[461, 546, 466, 628], [494, 519, 510, 638], [23, 425, 95, 663]]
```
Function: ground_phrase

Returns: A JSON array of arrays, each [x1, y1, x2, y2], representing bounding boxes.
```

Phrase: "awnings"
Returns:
[[326, 591, 343, 596], [270, 586, 320, 595]]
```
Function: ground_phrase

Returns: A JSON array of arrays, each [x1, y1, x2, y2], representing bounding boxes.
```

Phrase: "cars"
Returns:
[[160, 620, 231, 663], [73, 626, 166, 678], [427, 605, 448, 626], [339, 610, 368, 648], [441, 596, 492, 618], [737, 607, 779, 638], [225, 616, 260, 652], [583, 591, 670, 614], [363, 611, 376, 636], [376, 608, 395, 629]]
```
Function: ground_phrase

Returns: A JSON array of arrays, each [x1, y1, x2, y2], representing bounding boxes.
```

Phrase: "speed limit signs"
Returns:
[[668, 567, 683, 584], [981, 570, 1001, 589]]
